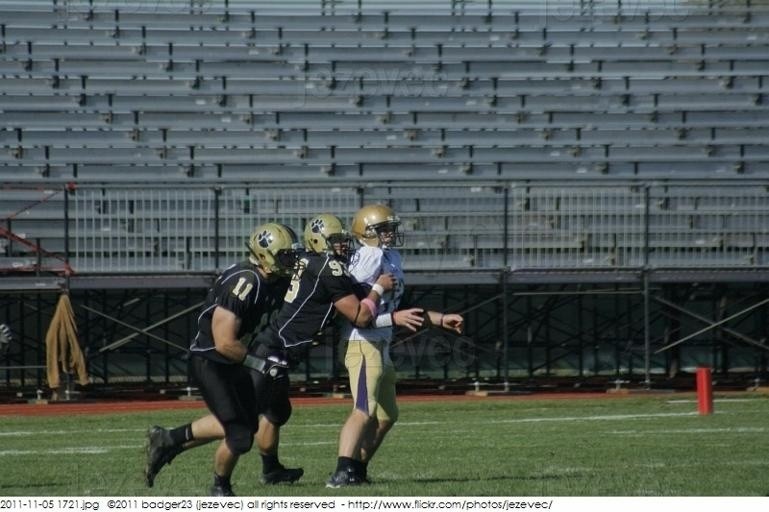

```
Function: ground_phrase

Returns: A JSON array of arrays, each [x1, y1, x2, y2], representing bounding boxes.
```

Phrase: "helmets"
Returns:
[[245, 223, 305, 278], [303, 214, 359, 272], [353, 204, 404, 247]]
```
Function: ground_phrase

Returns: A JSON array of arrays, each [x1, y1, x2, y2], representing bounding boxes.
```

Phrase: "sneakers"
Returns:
[[327, 469, 369, 489], [145, 426, 173, 488], [261, 466, 303, 484], [211, 482, 236, 496]]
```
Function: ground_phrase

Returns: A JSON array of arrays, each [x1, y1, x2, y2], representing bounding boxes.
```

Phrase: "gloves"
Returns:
[[244, 353, 289, 384]]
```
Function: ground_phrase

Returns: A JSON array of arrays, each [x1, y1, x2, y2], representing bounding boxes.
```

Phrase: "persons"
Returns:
[[167, 214, 400, 484], [146, 222, 305, 496], [325, 205, 464, 489]]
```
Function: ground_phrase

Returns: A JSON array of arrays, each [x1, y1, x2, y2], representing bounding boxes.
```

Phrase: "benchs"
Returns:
[[0, 1, 769, 271]]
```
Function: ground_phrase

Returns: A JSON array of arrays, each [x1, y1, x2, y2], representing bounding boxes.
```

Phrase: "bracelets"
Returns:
[[439, 311, 447, 329], [371, 311, 395, 327], [372, 282, 385, 295]]
